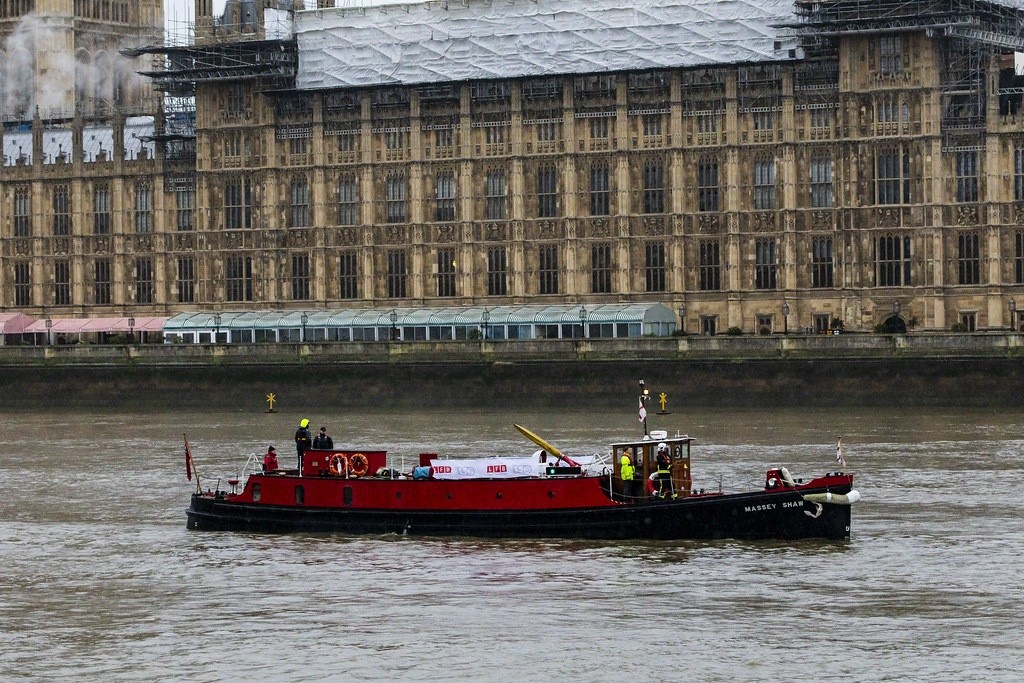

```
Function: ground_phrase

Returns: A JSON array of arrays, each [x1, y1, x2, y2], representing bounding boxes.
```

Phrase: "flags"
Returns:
[[638, 398, 647, 423], [836, 445, 846, 466], [184, 444, 192, 481]]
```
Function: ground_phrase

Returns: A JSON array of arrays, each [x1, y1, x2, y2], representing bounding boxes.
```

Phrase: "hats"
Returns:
[[269, 445, 275, 452]]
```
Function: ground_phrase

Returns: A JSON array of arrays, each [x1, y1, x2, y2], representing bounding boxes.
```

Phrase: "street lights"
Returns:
[[1009, 297, 1016, 332], [782, 302, 790, 335], [679, 303, 687, 336], [482, 308, 491, 340], [214, 312, 222, 343], [579, 304, 587, 338], [894, 299, 900, 333], [390, 310, 397, 340], [127, 314, 136, 344], [45, 316, 53, 345], [301, 311, 308, 342]]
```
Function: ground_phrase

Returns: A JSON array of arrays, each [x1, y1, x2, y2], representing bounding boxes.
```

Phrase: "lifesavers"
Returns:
[[329, 453, 349, 477], [647, 471, 671, 498], [349, 453, 369, 477]]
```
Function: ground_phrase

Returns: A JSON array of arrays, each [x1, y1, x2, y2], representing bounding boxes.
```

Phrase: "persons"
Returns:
[[313, 427, 333, 449], [295, 418, 313, 470], [264, 445, 278, 470], [657, 446, 678, 501], [620, 445, 635, 504]]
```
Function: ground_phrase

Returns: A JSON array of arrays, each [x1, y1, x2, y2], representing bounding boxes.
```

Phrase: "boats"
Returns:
[[184, 379, 862, 541]]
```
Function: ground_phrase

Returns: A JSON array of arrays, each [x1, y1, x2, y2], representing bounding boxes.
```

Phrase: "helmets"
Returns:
[[300, 418, 310, 428], [657, 443, 669, 452]]
